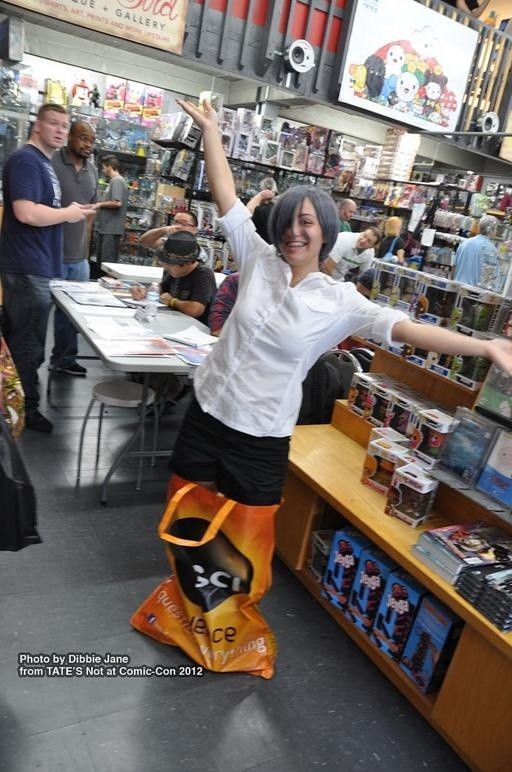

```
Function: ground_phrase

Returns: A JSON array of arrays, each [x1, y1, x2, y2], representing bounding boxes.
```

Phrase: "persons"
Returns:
[[139, 209, 209, 280], [170, 95, 511, 673], [0, 102, 93, 435], [208, 271, 241, 338], [337, 198, 357, 234], [377, 217, 406, 266], [245, 176, 281, 249], [128, 229, 216, 418], [321, 227, 383, 284], [449, 215, 503, 292], [51, 118, 97, 377], [96, 153, 129, 280]]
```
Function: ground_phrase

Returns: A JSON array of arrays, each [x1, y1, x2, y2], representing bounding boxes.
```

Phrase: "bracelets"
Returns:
[[170, 298, 178, 308]]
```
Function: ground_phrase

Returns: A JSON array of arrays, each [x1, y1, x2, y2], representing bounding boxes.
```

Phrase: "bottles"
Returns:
[[145, 281, 160, 318]]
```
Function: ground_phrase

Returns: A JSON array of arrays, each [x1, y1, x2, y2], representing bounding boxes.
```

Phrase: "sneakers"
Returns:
[[382, 251, 403, 273], [47, 354, 87, 377], [23, 407, 54, 433]]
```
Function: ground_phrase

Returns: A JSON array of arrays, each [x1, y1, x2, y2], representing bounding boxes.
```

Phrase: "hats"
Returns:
[[157, 230, 201, 266]]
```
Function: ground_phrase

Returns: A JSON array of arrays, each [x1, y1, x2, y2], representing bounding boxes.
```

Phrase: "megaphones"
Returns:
[[473, 111, 500, 140], [284, 38, 315, 74]]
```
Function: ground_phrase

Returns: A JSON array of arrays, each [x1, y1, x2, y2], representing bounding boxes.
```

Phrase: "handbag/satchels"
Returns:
[[128, 473, 284, 681]]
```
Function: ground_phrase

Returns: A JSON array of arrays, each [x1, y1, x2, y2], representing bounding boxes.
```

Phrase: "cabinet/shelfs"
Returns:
[[0, 103, 512, 772]]
